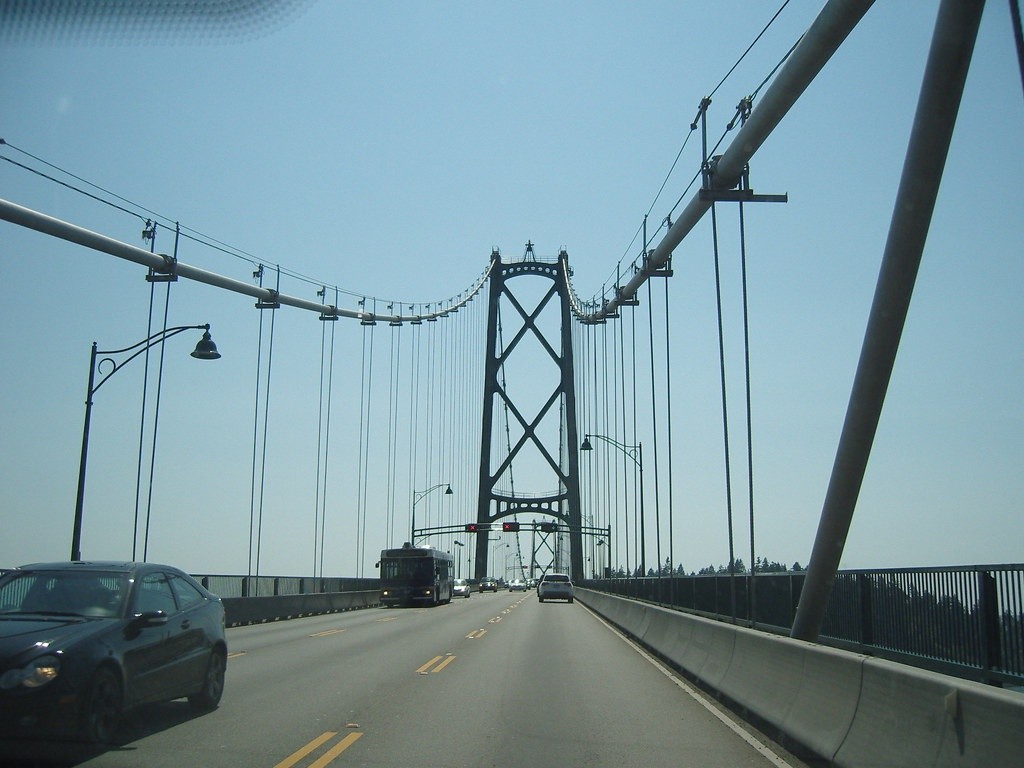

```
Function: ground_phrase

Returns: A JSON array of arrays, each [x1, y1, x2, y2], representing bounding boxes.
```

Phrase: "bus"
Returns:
[[374, 548, 456, 607]]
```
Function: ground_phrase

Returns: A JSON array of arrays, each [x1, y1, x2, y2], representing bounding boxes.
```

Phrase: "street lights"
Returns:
[[69, 322, 220, 576], [513, 556, 525, 580], [493, 543, 510, 579], [411, 483, 453, 549], [580, 433, 647, 577], [503, 552, 518, 581], [565, 511, 595, 580]]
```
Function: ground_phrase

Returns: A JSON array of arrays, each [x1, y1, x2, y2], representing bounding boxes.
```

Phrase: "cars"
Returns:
[[479, 577, 498, 593], [0, 560, 231, 753], [505, 574, 545, 594], [451, 578, 471, 599], [539, 575, 574, 605]]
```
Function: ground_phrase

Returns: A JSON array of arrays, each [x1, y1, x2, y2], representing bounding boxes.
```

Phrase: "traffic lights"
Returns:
[[503, 523, 520, 533], [465, 524, 478, 533], [542, 524, 558, 533]]
